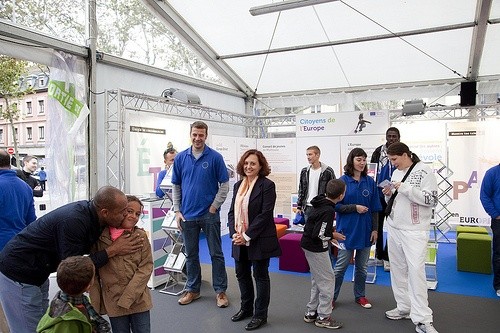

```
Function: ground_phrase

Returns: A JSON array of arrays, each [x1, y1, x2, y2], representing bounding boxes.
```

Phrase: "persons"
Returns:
[[11, 154, 17, 167], [383, 142, 439, 333], [367, 127, 401, 271], [0, 185, 146, 333], [88, 194, 154, 333], [479, 163, 500, 297], [36, 255, 112, 333], [13, 154, 43, 197], [171, 120, 230, 308], [156, 148, 179, 198], [333, 147, 383, 309], [38, 167, 46, 191], [0, 148, 38, 333], [228, 149, 282, 331], [297, 145, 336, 225], [299, 179, 346, 329]]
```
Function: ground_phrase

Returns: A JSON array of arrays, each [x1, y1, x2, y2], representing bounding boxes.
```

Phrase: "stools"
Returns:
[[455, 225, 492, 275], [274, 217, 289, 238], [279, 233, 310, 274]]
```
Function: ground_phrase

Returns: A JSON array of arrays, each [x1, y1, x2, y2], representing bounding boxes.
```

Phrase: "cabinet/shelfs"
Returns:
[[423, 206, 438, 291], [428, 157, 458, 244], [159, 184, 189, 296], [352, 162, 378, 283]]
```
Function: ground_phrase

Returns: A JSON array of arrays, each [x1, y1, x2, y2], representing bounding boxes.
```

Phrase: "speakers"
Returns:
[[461, 80, 476, 107]]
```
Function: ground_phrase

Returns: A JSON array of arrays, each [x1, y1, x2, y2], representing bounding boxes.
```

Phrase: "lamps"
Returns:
[[164, 87, 203, 105], [249, 0, 340, 16], [402, 101, 427, 116]]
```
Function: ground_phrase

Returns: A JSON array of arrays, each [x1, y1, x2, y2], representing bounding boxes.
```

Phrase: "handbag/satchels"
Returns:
[[385, 193, 396, 216]]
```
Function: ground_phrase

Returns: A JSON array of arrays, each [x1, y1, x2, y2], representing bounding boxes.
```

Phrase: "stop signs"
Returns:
[[8, 149, 14, 154]]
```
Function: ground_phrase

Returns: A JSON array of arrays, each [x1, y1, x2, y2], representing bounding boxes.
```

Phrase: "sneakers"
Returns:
[[315, 315, 342, 329], [415, 322, 439, 333], [355, 295, 372, 308], [303, 310, 319, 322], [385, 308, 411, 320], [332, 298, 335, 309], [216, 291, 229, 308], [178, 290, 201, 305]]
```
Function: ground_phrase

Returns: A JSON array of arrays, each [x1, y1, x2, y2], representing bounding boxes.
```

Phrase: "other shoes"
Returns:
[[496, 290, 500, 296]]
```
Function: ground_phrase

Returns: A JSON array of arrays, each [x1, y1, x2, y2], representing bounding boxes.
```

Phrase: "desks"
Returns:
[[139, 197, 174, 291]]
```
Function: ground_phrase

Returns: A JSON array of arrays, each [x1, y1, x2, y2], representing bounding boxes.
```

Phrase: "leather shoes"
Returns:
[[244, 316, 269, 330], [231, 308, 254, 321]]
